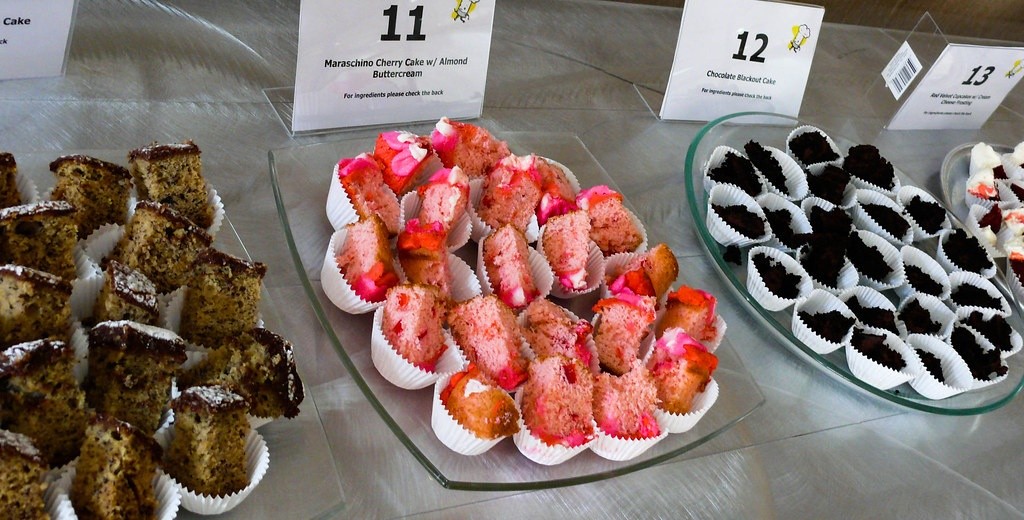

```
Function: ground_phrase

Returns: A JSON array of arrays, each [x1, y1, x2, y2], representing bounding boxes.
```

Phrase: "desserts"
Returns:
[[319, 116, 728, 462], [703, 125, 1024, 400], [964, 141, 1024, 303], [0, 139, 307, 520]]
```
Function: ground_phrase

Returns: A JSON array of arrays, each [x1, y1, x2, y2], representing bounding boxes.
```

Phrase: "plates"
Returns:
[[268, 131, 767, 491], [683, 112, 1024, 414], [17, 149, 347, 520], [940, 142, 1014, 222]]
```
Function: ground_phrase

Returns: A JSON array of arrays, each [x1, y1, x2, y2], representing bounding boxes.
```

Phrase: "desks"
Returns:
[[0, 0, 1024, 520]]
